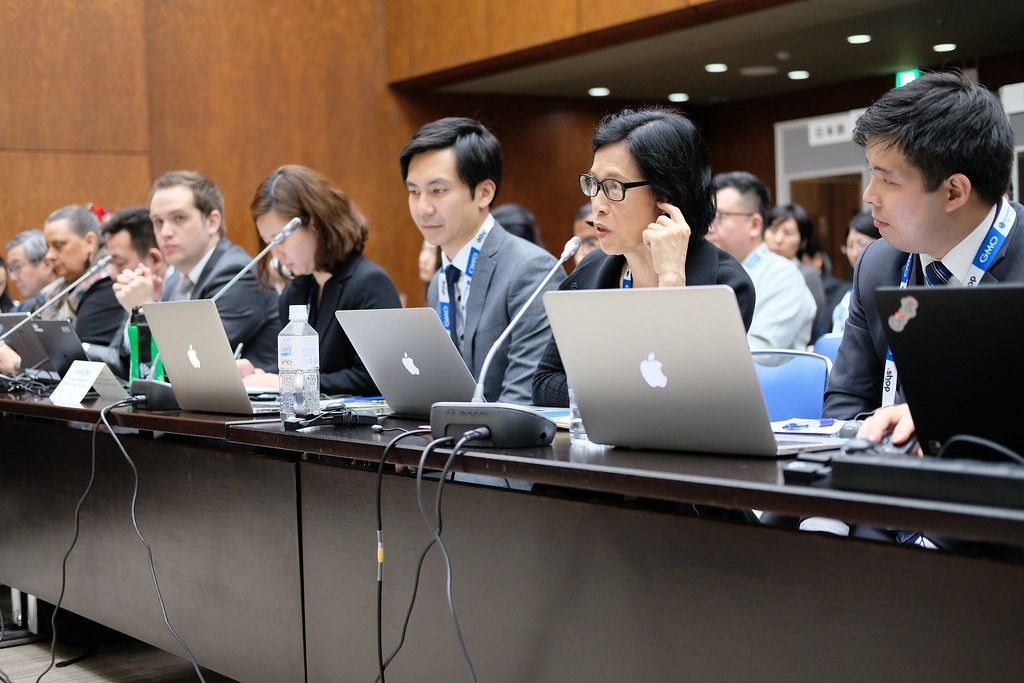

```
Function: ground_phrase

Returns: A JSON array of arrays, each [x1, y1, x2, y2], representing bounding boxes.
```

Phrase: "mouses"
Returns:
[[870, 430, 920, 455]]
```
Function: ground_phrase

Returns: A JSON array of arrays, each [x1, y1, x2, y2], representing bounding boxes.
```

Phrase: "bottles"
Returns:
[[567, 383, 604, 447], [276, 304, 323, 432], [127, 305, 166, 396]]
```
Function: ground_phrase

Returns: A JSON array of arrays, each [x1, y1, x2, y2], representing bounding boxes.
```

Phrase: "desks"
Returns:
[[0, 378, 1024, 683]]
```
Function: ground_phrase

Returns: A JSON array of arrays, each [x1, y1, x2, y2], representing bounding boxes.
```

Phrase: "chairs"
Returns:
[[751, 347, 833, 426]]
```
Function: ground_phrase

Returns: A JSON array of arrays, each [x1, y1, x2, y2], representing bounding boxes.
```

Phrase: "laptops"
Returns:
[[542, 286, 855, 456], [143, 299, 282, 415], [0, 313, 99, 394], [872, 283, 1024, 465], [334, 307, 488, 416]]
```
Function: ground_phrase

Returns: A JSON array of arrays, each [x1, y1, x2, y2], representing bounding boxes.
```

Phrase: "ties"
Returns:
[[179, 277, 192, 298], [926, 260, 954, 287], [446, 265, 461, 348]]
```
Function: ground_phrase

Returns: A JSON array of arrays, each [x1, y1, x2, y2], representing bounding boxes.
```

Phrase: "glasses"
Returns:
[[8, 255, 43, 277], [707, 212, 759, 233], [579, 171, 652, 202]]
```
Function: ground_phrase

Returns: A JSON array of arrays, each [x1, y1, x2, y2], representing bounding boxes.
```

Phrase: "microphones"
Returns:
[[430, 235, 583, 449], [130, 216, 301, 411], [0, 255, 113, 342]]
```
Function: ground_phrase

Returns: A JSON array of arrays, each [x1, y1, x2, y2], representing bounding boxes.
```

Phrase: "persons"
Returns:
[[112, 170, 282, 374], [0, 205, 125, 375], [531, 111, 756, 407], [236, 164, 403, 396], [824, 212, 882, 331], [573, 203, 600, 266], [762, 203, 838, 324], [83, 207, 169, 379], [419, 239, 443, 283], [825, 72, 1024, 444], [705, 170, 818, 365], [399, 117, 568, 401]]
